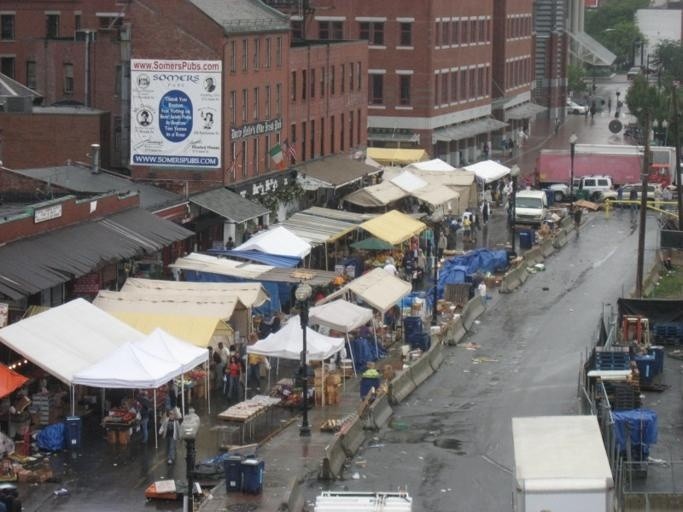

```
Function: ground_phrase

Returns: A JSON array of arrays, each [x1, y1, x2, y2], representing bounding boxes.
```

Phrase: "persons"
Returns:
[[200, 112, 214, 131], [477, 123, 542, 192], [138, 112, 150, 128], [614, 180, 678, 216], [118, 380, 201, 445], [311, 250, 426, 304], [203, 340, 263, 400], [0, 377, 70, 440], [382, 180, 513, 284], [546, 204, 584, 231], [158, 409, 182, 464], [202, 77, 216, 94], [360, 368, 380, 400]]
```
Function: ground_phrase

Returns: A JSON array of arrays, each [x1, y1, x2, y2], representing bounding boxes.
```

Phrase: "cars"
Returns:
[[626, 66, 645, 81], [567, 99, 590, 115]]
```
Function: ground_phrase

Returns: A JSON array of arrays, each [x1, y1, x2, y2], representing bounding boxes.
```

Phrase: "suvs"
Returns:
[[547, 173, 616, 204], [598, 181, 668, 208]]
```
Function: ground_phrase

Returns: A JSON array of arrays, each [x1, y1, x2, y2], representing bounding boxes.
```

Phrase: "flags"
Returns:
[[268, 139, 296, 171]]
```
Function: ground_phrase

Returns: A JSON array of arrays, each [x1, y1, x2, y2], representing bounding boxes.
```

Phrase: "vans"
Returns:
[[508, 188, 549, 231]]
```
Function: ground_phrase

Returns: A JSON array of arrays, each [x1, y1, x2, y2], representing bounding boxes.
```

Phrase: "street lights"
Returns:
[[294, 281, 315, 438], [179, 403, 199, 512], [430, 207, 444, 328], [508, 162, 521, 255], [567, 131, 579, 216]]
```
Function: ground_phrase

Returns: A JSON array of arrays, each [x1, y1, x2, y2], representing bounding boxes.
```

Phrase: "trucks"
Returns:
[[534, 148, 673, 194]]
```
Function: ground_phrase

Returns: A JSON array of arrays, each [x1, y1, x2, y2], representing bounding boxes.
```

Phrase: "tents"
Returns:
[[286, 299, 380, 378], [245, 316, 346, 409], [315, 267, 413, 350]]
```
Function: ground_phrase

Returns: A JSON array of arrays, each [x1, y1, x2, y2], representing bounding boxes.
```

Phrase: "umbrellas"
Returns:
[[348, 236, 393, 251]]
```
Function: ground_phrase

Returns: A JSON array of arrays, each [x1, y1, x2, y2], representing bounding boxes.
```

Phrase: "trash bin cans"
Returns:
[[544, 188, 554, 207], [360, 377, 382, 400], [224, 453, 265, 495], [520, 232, 530, 248], [65, 416, 80, 450], [404, 316, 431, 352], [636, 345, 666, 382]]
